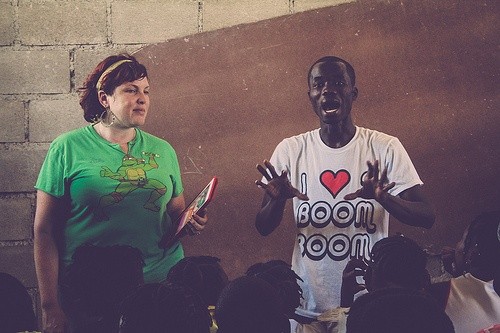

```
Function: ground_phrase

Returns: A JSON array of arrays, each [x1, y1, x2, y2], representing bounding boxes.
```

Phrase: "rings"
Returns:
[[197, 232, 200, 235]]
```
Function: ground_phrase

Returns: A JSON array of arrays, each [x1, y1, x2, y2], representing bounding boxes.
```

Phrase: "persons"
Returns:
[[255, 56, 436, 333], [339, 214, 500, 333], [0, 242, 303, 333], [34, 53, 208, 333]]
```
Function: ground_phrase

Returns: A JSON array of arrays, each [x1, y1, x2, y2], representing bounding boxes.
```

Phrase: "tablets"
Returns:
[[168, 175, 217, 244]]
